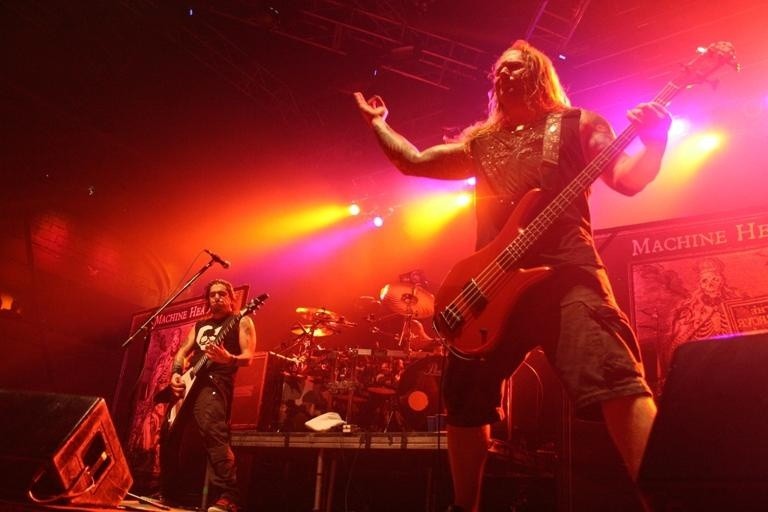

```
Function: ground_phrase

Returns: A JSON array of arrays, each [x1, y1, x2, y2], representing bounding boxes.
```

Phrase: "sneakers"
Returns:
[[140, 491, 176, 506], [208, 497, 243, 511]]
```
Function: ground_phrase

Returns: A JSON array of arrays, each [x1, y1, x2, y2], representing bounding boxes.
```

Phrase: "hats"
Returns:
[[696, 258, 724, 273]]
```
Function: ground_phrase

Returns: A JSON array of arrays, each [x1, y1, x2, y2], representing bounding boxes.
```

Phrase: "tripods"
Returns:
[[383, 360, 415, 433], [120, 263, 219, 510]]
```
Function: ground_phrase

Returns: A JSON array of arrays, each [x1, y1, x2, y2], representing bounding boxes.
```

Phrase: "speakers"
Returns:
[[230, 351, 297, 432], [0, 389, 134, 512], [637, 328, 768, 512]]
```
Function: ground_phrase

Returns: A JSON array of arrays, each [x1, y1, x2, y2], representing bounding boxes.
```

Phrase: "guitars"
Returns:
[[432, 39, 739, 363], [153, 292, 269, 433]]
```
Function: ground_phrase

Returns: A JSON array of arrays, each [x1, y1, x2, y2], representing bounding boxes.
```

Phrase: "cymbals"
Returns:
[[291, 326, 336, 336], [296, 306, 339, 323], [381, 284, 436, 319]]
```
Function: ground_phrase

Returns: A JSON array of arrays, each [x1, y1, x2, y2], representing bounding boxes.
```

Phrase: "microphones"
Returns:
[[203, 248, 231, 269]]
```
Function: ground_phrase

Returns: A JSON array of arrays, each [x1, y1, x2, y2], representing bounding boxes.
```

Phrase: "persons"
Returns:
[[171, 279, 256, 512], [353, 40, 672, 512]]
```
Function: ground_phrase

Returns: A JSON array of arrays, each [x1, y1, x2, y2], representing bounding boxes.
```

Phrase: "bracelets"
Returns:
[[172, 364, 183, 374], [222, 354, 238, 368]]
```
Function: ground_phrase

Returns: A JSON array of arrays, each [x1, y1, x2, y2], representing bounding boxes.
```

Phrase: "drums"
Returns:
[[280, 355, 309, 376], [320, 350, 352, 389], [397, 355, 447, 431], [361, 358, 404, 394]]
